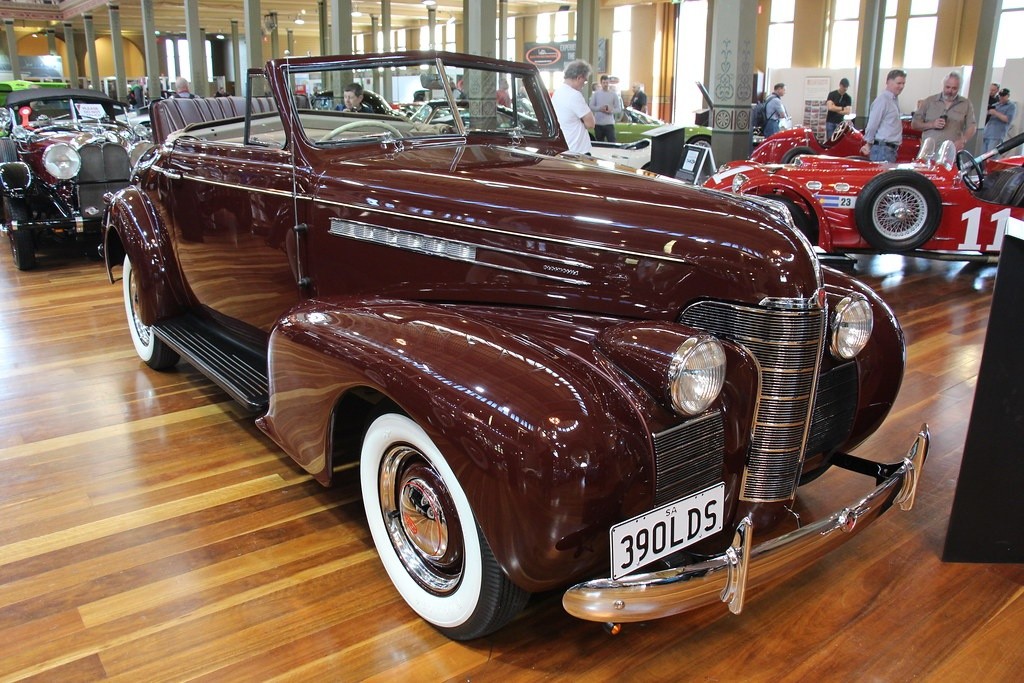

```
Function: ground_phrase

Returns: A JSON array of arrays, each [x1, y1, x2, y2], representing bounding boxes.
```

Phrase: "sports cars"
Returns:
[[748, 112, 922, 164], [101, 49, 930, 643], [0, 88, 156, 271], [702, 137, 1024, 264], [585, 107, 713, 149]]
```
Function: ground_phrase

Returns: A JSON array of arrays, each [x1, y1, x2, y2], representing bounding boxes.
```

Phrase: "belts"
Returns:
[[874, 140, 899, 149]]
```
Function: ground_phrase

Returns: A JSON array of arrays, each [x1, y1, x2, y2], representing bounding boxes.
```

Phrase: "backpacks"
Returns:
[[750, 94, 779, 128]]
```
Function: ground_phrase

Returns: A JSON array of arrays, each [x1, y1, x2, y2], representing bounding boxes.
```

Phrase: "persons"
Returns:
[[448, 76, 464, 91], [986, 84, 1017, 160], [310, 88, 320, 106], [762, 83, 786, 138], [168, 78, 200, 98], [495, 78, 512, 108], [826, 78, 852, 142], [549, 60, 596, 155], [343, 83, 374, 113], [127, 80, 145, 107], [216, 88, 231, 97], [911, 72, 977, 160], [589, 74, 620, 142], [859, 70, 907, 163], [628, 81, 647, 123]]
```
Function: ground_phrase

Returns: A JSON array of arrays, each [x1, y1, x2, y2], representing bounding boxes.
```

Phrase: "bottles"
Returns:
[[937, 109, 947, 130]]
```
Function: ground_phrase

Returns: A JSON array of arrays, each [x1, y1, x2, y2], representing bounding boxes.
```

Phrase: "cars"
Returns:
[[410, 99, 542, 135]]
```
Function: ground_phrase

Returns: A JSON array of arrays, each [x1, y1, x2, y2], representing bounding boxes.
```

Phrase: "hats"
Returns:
[[840, 78, 850, 87], [999, 88, 1010, 97]]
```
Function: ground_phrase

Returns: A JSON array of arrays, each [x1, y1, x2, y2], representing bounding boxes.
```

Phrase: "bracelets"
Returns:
[[842, 107, 844, 111]]
